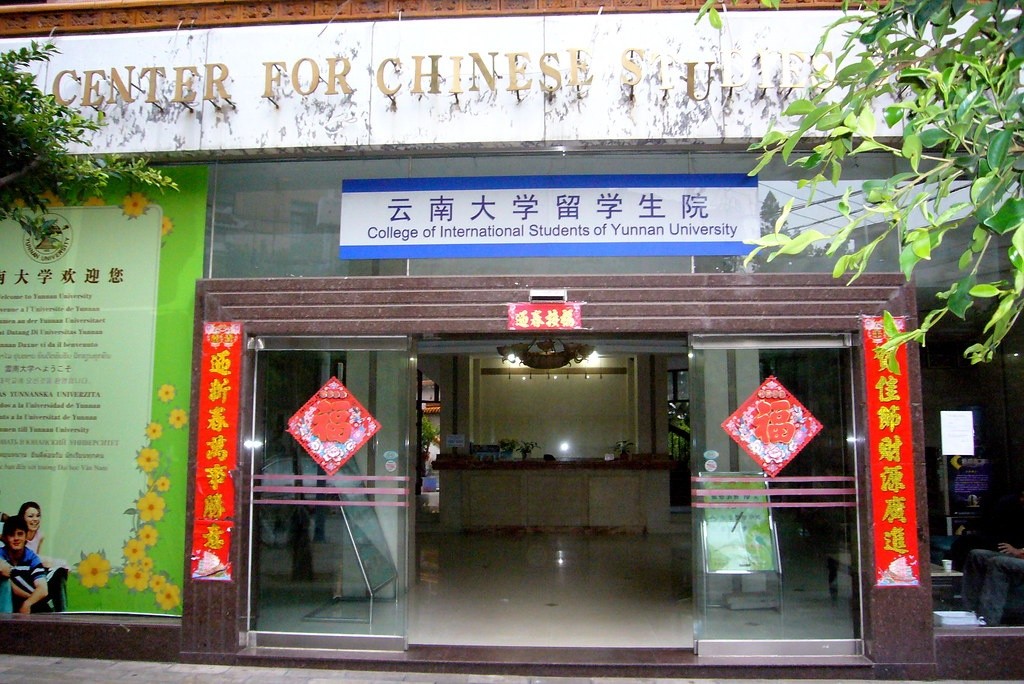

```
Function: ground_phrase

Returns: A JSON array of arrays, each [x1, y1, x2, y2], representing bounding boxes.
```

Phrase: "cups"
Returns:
[[941, 559, 952, 572]]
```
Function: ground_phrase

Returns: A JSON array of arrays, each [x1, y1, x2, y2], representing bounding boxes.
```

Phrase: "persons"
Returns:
[[990, 485, 1024, 543], [0, 501, 71, 614], [962, 543, 1024, 626]]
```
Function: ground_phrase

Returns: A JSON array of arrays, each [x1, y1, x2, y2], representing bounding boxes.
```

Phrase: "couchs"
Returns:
[[936, 534, 1024, 627]]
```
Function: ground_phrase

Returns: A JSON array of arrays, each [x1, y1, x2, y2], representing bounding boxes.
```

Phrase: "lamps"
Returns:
[[497, 337, 596, 370]]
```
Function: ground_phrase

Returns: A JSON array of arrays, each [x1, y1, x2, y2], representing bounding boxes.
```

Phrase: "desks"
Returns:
[[827, 553, 964, 639]]
